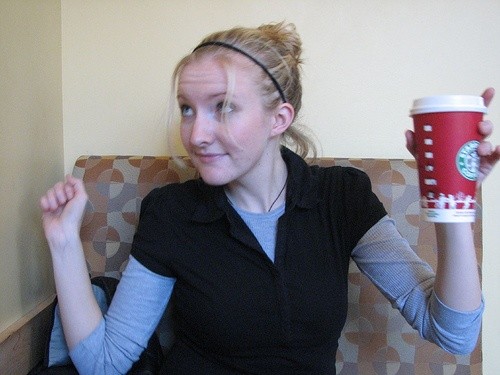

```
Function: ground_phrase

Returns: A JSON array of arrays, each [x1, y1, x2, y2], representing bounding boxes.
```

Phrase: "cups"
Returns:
[[409, 96, 489, 224]]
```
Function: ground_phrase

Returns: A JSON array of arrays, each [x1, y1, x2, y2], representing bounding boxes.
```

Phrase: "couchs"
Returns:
[[36, 153, 484, 375]]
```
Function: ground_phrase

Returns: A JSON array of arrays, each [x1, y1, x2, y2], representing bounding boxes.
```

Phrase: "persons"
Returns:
[[37, 22, 485, 375]]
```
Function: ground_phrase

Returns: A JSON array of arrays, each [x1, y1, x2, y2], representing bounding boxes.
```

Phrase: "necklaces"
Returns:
[[266, 175, 289, 216]]
